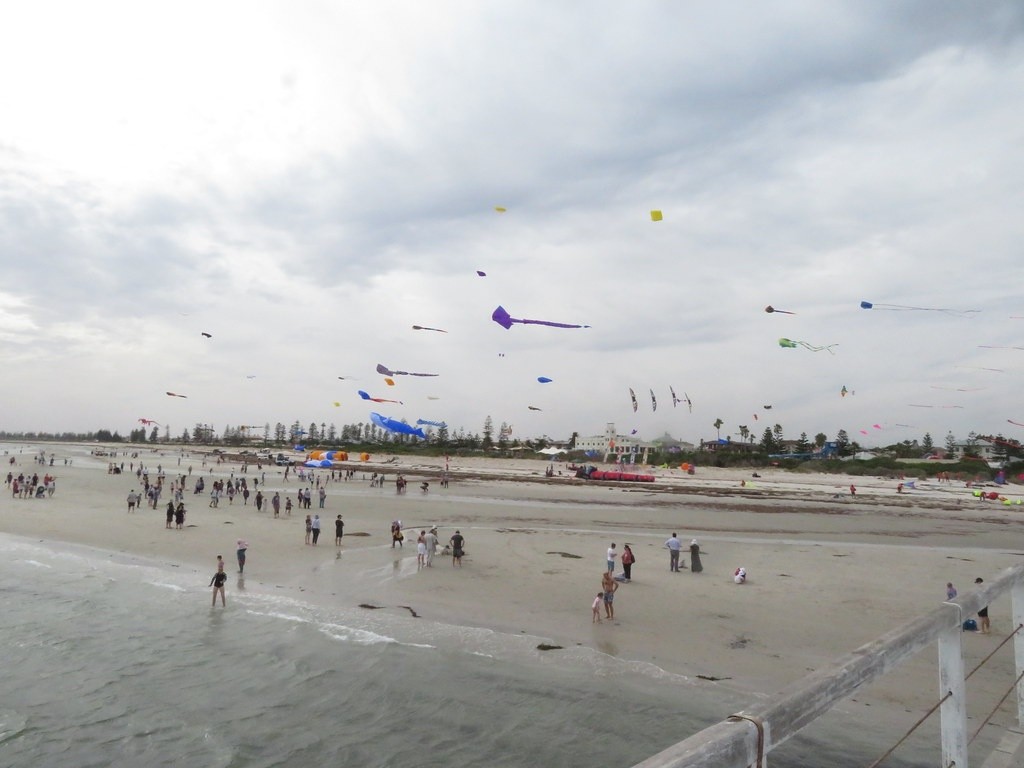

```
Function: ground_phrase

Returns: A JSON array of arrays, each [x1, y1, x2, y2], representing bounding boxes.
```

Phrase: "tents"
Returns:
[[486, 446, 568, 461]]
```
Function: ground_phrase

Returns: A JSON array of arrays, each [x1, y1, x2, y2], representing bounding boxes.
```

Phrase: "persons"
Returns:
[[391, 520, 404, 548], [936, 470, 986, 502], [591, 592, 603, 623], [897, 483, 905, 493], [335, 514, 345, 546], [208, 555, 227, 607], [325, 454, 449, 492], [620, 545, 631, 584], [417, 525, 465, 568], [607, 543, 617, 576], [946, 583, 957, 599], [850, 484, 856, 498], [90, 447, 327, 519], [665, 532, 681, 573], [975, 577, 990, 634], [690, 539, 703, 573], [305, 514, 321, 545], [602, 572, 619, 621], [3, 445, 73, 498], [165, 502, 187, 530], [734, 567, 747, 583], [236, 536, 249, 572]]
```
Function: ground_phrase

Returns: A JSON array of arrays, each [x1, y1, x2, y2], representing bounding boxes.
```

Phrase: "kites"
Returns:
[[128, 195, 1024, 451]]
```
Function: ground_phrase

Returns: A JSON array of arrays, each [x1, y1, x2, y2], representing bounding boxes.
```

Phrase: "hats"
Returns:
[[314, 514, 319, 519], [691, 539, 697, 544]]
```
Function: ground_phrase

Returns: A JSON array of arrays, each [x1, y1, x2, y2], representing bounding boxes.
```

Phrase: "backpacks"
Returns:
[[963, 619, 977, 632]]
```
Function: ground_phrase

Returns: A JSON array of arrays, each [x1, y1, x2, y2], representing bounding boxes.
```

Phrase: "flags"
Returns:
[[609, 441, 615, 448]]
